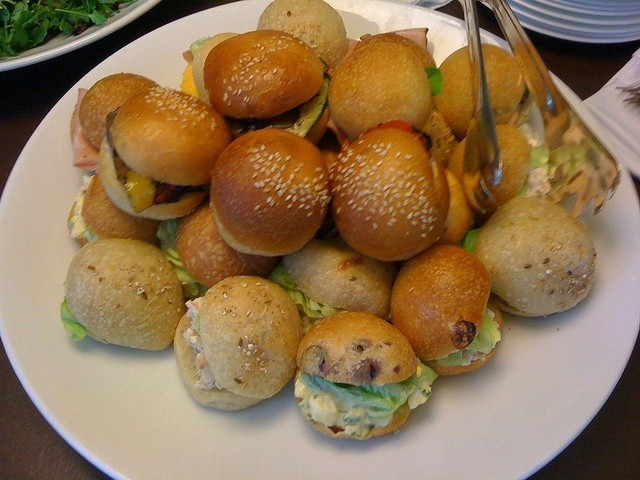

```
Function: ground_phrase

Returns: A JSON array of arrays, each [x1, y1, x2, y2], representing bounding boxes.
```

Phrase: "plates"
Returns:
[[0, 0, 160, 72], [482, 0, 640, 44], [2, 0, 640, 476]]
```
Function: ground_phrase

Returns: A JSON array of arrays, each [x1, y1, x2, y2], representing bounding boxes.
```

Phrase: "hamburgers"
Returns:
[[61, 0, 597, 441]]
